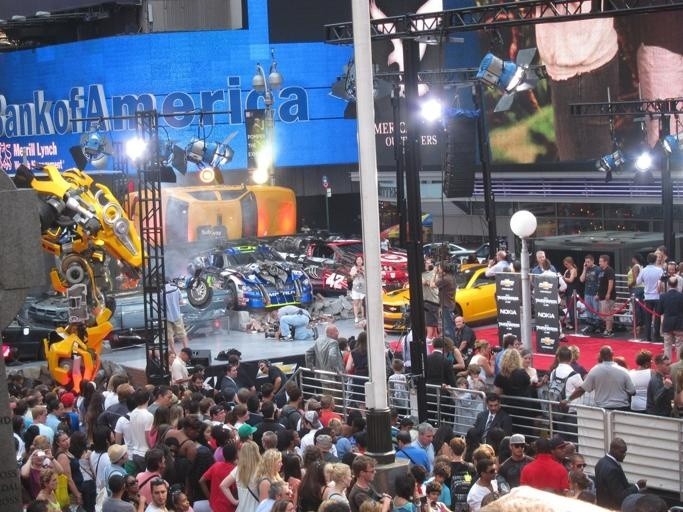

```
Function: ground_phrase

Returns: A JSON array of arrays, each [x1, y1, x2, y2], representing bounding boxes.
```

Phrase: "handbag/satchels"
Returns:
[[55, 474, 70, 507], [94, 486, 107, 512]]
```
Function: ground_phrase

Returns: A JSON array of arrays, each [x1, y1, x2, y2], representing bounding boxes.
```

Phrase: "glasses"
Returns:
[[577, 464, 586, 468]]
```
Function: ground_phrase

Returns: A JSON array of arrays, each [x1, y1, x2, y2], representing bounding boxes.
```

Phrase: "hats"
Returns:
[[181, 348, 193, 358], [304, 411, 323, 430], [61, 392, 74, 406], [238, 424, 258, 437], [550, 437, 569, 449], [510, 434, 526, 444], [108, 444, 128, 463], [315, 435, 333, 452]]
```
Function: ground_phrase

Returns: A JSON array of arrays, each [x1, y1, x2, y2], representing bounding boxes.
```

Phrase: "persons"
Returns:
[[159, 280, 188, 357], [424, 247, 683, 443], [350, 256, 366, 327], [6, 302, 648, 512]]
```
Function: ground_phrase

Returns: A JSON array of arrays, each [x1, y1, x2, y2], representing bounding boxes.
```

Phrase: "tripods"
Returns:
[[393, 324, 416, 373]]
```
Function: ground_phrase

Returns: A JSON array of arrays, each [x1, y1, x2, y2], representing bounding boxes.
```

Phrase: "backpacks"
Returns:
[[278, 409, 294, 428], [450, 463, 471, 512], [549, 369, 576, 420]]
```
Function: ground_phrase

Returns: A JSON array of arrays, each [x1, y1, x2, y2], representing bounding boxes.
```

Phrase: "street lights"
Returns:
[[250, 48, 283, 187], [509, 209, 537, 367]]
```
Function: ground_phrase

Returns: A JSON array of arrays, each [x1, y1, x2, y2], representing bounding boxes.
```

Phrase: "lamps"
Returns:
[[85, 49, 284, 185], [596, 103, 683, 174], [329, 42, 550, 110]]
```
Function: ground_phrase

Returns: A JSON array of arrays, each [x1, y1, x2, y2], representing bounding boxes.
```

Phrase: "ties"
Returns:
[[483, 416, 493, 442]]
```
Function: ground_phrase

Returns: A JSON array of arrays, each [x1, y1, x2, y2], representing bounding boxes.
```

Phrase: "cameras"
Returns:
[[37, 449, 45, 456]]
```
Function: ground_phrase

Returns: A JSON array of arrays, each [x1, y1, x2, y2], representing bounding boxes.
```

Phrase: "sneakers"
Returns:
[[601, 329, 614, 337], [280, 336, 293, 341], [312, 327, 318, 340]]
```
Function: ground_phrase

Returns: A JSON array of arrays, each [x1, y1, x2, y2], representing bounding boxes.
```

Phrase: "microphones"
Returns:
[[358, 263, 362, 271]]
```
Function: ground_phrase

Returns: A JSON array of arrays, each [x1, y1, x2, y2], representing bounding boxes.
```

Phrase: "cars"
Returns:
[[383, 264, 496, 334], [0, 228, 489, 362], [121, 183, 297, 245]]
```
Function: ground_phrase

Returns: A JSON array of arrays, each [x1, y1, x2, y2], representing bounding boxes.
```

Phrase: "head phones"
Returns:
[[666, 261, 679, 272]]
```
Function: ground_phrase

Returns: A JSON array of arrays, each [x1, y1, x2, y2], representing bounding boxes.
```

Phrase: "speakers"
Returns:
[[190, 349, 211, 366], [443, 118, 476, 198]]
